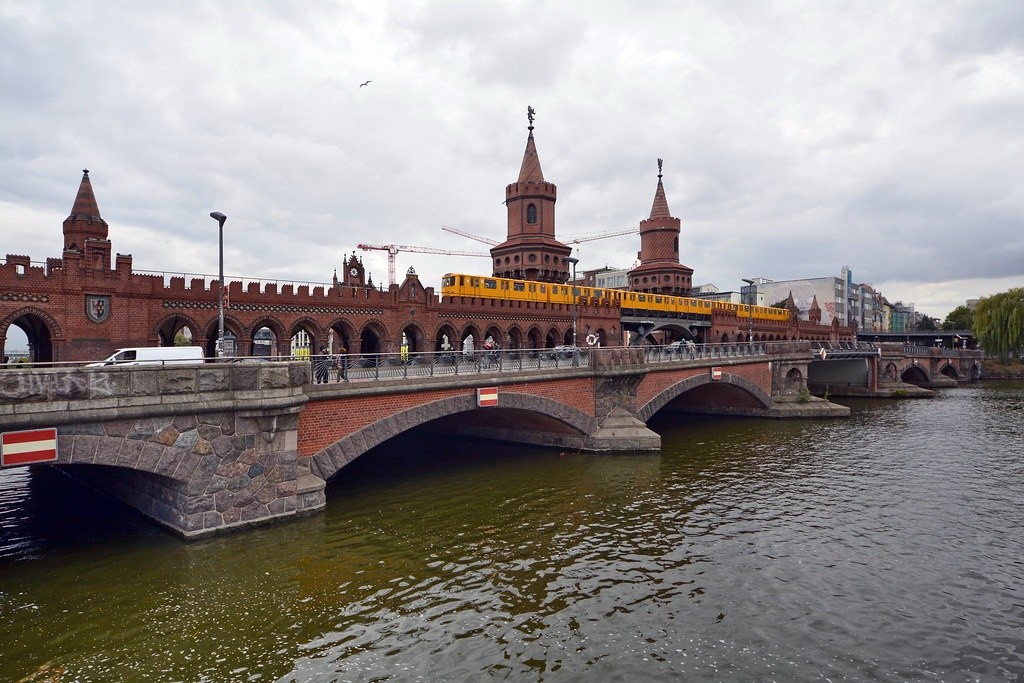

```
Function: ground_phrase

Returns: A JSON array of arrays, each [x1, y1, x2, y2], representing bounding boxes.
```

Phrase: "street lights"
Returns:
[[742, 278, 755, 355], [209, 211, 227, 363], [562, 256, 580, 367], [947, 320, 956, 357], [848, 297, 859, 333], [933, 317, 941, 355]]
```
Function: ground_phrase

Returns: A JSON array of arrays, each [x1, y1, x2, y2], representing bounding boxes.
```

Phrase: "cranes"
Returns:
[[441, 225, 640, 252], [356, 242, 492, 284]]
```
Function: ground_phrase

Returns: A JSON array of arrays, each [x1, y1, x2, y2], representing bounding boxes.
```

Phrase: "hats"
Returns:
[[489, 339, 493, 343], [320, 345, 327, 350]]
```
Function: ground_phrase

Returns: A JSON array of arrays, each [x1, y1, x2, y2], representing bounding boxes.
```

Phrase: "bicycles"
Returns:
[[474, 346, 501, 373]]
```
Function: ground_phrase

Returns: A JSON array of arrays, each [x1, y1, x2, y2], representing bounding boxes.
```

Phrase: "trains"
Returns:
[[441, 272, 791, 322]]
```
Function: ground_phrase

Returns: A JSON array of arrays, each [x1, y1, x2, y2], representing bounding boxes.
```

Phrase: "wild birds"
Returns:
[[360, 81, 373, 87]]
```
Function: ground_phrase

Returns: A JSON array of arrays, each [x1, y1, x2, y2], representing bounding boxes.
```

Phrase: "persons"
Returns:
[[316, 345, 329, 384], [336, 348, 351, 383], [678, 339, 690, 361], [688, 341, 696, 360]]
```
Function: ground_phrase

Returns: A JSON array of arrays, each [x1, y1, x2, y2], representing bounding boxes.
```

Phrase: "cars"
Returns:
[[545, 345, 582, 361], [664, 341, 696, 354], [223, 358, 270, 364]]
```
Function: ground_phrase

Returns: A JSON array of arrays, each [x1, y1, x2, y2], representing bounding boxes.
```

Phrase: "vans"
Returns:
[[84, 346, 206, 367]]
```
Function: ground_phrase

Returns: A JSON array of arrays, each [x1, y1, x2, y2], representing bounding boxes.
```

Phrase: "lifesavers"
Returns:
[[585, 334, 597, 346]]
